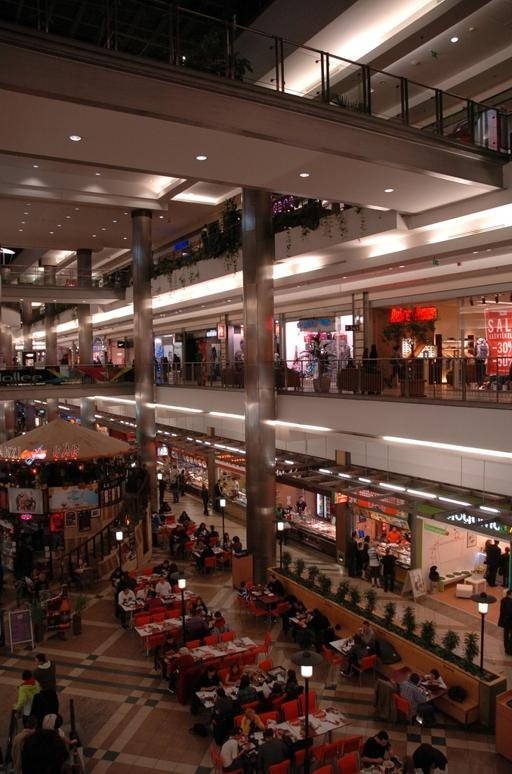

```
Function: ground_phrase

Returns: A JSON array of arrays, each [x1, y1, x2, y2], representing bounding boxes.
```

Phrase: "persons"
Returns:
[[91, 337, 488, 405], [8, 655, 78, 774], [108, 468, 512, 774]]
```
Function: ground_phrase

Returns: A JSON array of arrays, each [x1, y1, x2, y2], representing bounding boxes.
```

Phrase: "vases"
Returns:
[[314, 378, 330, 393]]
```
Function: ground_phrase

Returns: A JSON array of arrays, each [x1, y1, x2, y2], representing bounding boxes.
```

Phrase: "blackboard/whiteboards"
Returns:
[[409, 568, 427, 597], [9, 610, 33, 645]]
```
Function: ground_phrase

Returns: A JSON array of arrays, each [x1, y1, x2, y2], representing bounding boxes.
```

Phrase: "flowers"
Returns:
[[294, 330, 337, 376]]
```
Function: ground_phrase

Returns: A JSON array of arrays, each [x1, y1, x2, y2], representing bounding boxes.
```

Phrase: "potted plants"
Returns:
[[72, 595, 86, 634], [29, 598, 45, 642], [384, 319, 435, 397]]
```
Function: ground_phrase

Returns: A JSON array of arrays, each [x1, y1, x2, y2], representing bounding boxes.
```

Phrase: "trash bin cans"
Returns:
[[232, 549, 253, 589], [74, 614, 81, 633]]
